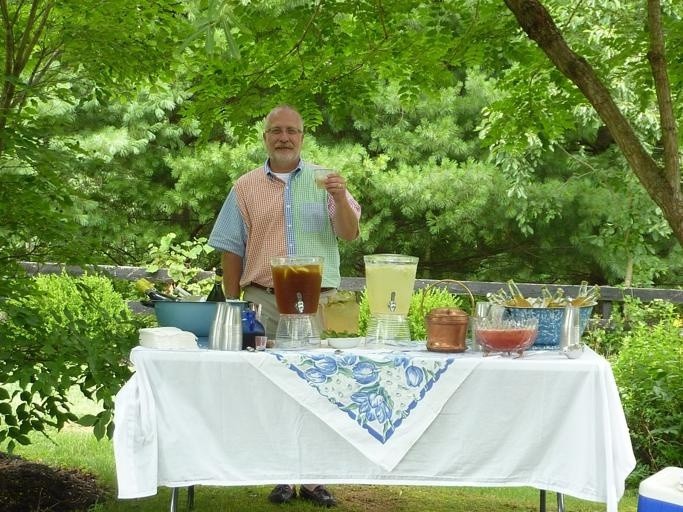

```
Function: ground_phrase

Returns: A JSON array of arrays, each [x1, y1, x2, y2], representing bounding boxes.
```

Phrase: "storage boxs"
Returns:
[[634, 465, 682, 512]]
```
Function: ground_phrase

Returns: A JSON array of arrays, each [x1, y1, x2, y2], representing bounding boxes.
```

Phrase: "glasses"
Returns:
[[265, 127, 297, 135]]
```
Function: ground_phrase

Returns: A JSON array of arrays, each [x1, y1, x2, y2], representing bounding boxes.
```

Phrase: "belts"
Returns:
[[251, 281, 335, 295]]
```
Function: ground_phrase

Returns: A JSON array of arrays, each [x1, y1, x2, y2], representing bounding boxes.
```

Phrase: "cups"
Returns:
[[254, 336, 267, 351], [558, 306, 583, 358], [313, 168, 333, 190]]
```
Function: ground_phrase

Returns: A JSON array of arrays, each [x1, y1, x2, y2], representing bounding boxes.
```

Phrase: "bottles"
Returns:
[[485, 278, 600, 308], [209, 304, 243, 351], [205, 272, 228, 301], [134, 276, 202, 301], [241, 300, 266, 351]]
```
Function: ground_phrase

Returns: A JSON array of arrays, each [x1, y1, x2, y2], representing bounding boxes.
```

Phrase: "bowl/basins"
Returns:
[[151, 299, 247, 347], [328, 336, 363, 349], [476, 322, 540, 359], [476, 298, 599, 349]]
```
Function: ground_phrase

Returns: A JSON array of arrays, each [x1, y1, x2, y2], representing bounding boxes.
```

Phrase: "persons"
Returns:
[[207, 106, 360, 507]]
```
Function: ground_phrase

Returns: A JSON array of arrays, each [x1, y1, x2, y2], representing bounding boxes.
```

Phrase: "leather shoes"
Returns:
[[299, 485, 338, 506], [269, 483, 297, 504]]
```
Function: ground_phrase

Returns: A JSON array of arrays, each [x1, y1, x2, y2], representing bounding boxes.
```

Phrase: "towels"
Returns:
[[135, 326, 200, 350]]
